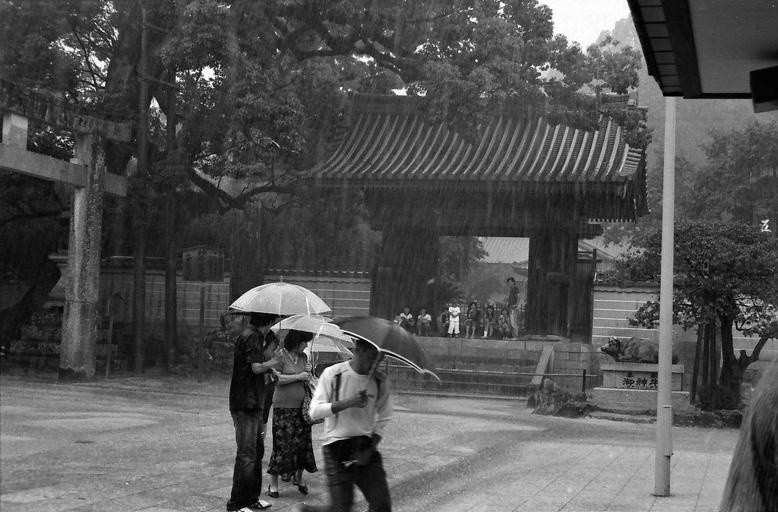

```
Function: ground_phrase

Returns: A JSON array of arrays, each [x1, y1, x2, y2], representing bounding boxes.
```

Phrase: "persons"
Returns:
[[261, 329, 318, 498], [400, 302, 512, 340], [308, 339, 392, 512], [507, 277, 520, 341], [227, 315, 282, 512]]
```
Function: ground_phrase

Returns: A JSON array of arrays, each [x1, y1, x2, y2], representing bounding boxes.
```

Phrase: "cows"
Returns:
[[600, 336, 678, 364]]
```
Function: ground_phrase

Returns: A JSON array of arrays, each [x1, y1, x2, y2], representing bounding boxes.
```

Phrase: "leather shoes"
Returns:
[[267, 484, 279, 498], [294, 473, 308, 495]]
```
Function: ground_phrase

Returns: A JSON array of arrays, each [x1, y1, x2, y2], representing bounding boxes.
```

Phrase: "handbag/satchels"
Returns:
[[301, 374, 325, 425]]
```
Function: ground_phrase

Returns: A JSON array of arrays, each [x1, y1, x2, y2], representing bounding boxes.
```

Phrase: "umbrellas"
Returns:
[[329, 316, 441, 394], [270, 315, 353, 361], [308, 334, 353, 361], [228, 275, 333, 353]]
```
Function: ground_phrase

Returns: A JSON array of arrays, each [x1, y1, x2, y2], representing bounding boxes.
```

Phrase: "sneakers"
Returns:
[[229, 506, 253, 512], [249, 499, 271, 509]]
